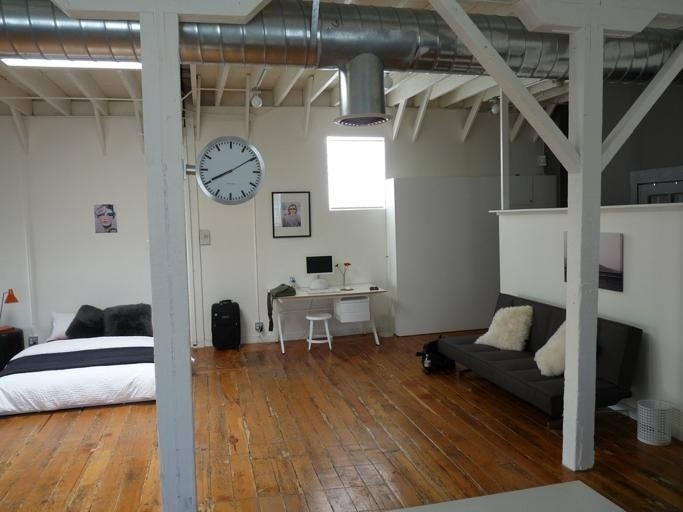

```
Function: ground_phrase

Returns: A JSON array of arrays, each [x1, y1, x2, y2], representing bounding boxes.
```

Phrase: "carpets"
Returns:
[[389, 478, 626, 512]]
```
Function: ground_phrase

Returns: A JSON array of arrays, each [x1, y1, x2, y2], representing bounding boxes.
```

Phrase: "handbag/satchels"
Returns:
[[267, 283, 297, 331], [415, 333, 457, 375]]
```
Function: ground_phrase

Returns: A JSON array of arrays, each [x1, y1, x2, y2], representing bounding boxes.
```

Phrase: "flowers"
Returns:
[[333, 261, 351, 273]]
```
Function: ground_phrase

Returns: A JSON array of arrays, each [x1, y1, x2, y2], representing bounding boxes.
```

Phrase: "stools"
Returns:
[[304, 311, 334, 352]]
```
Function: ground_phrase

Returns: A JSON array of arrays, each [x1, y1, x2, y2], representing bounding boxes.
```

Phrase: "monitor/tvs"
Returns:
[[305, 255, 334, 290]]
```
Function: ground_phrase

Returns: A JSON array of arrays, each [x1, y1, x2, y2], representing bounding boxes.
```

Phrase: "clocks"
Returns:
[[184, 135, 265, 206]]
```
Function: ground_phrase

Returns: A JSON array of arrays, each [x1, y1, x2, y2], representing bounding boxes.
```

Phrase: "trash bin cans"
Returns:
[[637, 399, 673, 446]]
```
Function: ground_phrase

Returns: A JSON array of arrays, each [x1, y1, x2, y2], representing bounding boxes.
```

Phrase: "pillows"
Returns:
[[46, 311, 76, 343], [475, 305, 536, 351], [101, 303, 153, 338], [533, 320, 566, 376], [65, 304, 104, 339]]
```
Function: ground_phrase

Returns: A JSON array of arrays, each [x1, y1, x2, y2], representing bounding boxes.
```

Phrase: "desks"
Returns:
[[270, 282, 388, 354]]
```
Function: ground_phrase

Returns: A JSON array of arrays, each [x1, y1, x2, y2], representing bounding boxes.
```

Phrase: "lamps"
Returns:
[[489, 96, 500, 115], [250, 88, 262, 109], [0, 287, 19, 331]]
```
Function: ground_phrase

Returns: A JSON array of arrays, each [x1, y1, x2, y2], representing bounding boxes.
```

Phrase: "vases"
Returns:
[[341, 273, 345, 290]]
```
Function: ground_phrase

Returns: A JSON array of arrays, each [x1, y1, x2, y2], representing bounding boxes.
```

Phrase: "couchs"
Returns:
[[436, 289, 643, 432]]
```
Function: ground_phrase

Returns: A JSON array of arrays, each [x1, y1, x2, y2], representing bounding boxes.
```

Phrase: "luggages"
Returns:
[[210, 298, 242, 350]]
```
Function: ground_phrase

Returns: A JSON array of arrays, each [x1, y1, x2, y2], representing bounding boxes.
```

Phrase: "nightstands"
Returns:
[[0, 326, 24, 371]]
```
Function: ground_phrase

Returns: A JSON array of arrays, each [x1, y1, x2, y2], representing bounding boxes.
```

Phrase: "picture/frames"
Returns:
[[271, 191, 311, 238]]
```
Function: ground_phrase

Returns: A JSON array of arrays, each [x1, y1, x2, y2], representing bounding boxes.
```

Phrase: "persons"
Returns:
[[95, 204, 117, 233], [282, 204, 301, 227]]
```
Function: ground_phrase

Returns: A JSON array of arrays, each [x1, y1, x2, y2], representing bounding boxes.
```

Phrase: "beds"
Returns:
[[0, 301, 197, 417]]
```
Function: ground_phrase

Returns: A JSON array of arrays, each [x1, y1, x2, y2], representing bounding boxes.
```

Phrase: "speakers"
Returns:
[[211, 304, 241, 350]]
